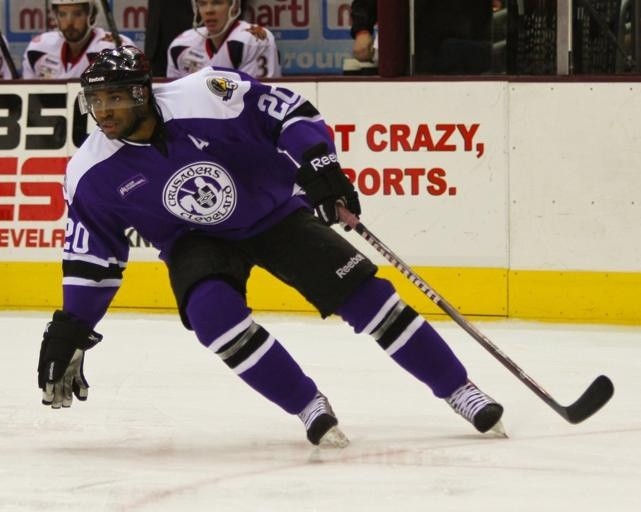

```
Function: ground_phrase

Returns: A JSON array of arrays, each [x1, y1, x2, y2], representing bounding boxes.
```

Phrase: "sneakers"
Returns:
[[298, 390, 339, 445], [444, 378, 505, 433]]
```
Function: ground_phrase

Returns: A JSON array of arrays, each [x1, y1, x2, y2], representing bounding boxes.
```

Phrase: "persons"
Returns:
[[37, 45, 504, 446], [165, 0, 282, 78], [350, 1, 506, 75], [22, 0, 137, 81]]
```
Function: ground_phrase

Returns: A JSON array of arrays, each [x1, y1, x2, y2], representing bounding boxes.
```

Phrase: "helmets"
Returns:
[[49, 0, 95, 19], [77, 45, 154, 115]]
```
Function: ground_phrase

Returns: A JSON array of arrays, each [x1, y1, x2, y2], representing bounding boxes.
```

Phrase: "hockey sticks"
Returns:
[[337, 202, 613, 423]]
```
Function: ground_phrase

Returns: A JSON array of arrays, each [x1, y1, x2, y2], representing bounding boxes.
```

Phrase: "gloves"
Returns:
[[38, 308, 104, 409], [293, 141, 362, 232]]
